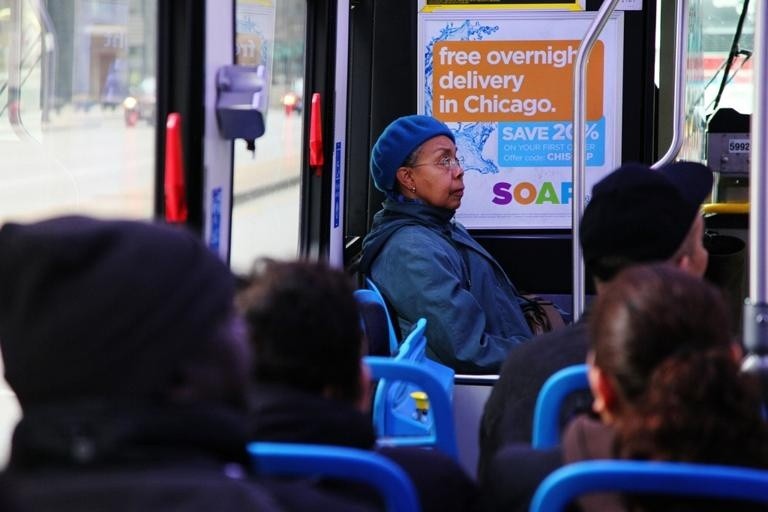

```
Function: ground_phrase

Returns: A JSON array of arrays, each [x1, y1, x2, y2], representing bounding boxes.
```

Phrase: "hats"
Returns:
[[579, 161, 713, 278], [0, 215, 239, 414], [369, 115, 456, 193]]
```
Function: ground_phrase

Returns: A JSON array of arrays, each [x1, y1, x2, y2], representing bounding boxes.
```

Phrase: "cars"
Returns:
[[124, 78, 156, 125]]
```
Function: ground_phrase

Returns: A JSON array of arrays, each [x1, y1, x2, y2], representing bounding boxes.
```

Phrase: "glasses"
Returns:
[[408, 157, 459, 170]]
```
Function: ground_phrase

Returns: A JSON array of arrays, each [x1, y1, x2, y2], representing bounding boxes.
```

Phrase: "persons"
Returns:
[[1, 216, 349, 511], [354, 116, 535, 385], [238, 253, 489, 510], [477, 162, 714, 477], [477, 261, 768, 511]]
